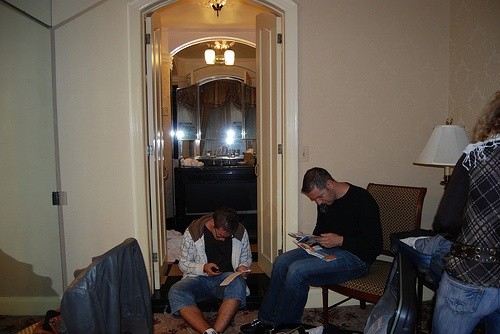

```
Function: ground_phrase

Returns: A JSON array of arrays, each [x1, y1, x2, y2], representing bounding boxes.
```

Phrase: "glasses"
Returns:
[[214, 227, 234, 240]]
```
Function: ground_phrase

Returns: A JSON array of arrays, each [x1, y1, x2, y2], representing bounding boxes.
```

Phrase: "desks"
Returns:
[[175, 167, 258, 231]]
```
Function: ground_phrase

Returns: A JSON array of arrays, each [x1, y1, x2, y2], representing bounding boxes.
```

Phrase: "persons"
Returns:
[[167, 208, 253, 334], [431, 87, 500, 334], [242, 167, 382, 334]]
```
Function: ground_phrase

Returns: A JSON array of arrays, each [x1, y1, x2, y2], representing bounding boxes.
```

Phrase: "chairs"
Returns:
[[323, 183, 427, 325], [18, 237, 153, 334]]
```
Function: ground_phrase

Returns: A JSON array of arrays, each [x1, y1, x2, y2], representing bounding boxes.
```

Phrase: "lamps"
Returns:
[[413, 118, 469, 186], [204, 41, 237, 66], [210, 0, 226, 17]]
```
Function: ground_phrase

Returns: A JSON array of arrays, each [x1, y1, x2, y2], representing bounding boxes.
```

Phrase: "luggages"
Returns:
[[390, 227, 454, 283], [305, 252, 417, 333]]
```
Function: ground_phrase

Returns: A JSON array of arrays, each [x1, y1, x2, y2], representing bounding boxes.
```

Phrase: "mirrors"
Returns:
[[171, 73, 256, 165]]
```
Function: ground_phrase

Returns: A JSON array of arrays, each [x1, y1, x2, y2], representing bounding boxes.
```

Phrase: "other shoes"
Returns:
[[270, 319, 302, 334], [239, 317, 271, 334]]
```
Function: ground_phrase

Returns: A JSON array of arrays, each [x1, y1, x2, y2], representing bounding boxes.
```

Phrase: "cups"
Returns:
[[244, 153, 253, 165]]
